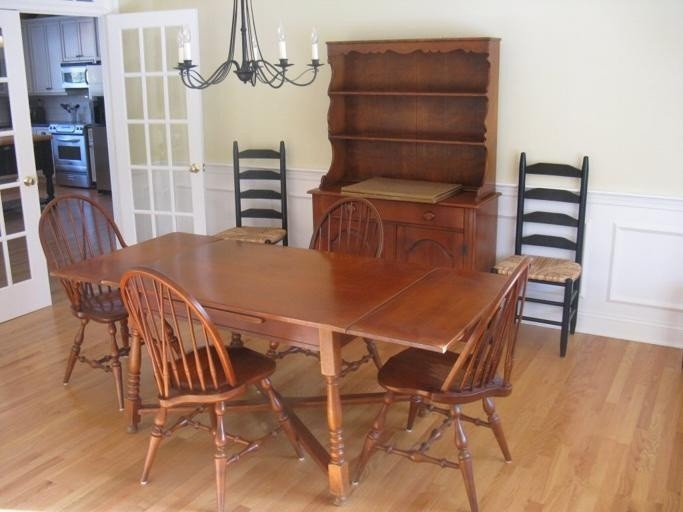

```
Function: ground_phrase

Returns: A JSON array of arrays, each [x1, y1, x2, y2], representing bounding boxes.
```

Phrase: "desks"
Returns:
[[46, 229, 515, 506], [0, 126, 57, 211]]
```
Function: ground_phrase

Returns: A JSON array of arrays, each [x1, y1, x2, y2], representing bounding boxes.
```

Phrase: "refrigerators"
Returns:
[[86, 65, 111, 194]]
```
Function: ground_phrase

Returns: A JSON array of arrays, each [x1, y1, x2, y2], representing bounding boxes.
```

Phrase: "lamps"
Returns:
[[177, 1, 325, 93]]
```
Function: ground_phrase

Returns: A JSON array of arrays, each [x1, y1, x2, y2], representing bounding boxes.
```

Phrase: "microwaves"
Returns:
[[60, 62, 98, 90]]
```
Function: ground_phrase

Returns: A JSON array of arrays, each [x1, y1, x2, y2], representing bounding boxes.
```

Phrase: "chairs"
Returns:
[[491, 151, 590, 358], [118, 267, 320, 509], [348, 254, 534, 512], [36, 192, 185, 413], [208, 137, 288, 247], [264, 193, 385, 375]]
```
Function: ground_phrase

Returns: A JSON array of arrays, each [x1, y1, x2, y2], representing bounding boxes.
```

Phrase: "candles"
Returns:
[[183, 42, 191, 60], [179, 47, 184, 63], [311, 42, 317, 59], [279, 40, 287, 58]]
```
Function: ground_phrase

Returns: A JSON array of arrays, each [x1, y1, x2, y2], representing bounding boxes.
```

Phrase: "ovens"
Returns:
[[50, 134, 90, 188]]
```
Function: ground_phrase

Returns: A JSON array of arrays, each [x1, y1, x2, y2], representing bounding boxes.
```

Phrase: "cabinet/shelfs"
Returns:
[[29, 20, 67, 94], [304, 34, 503, 278], [60, 16, 100, 62]]
